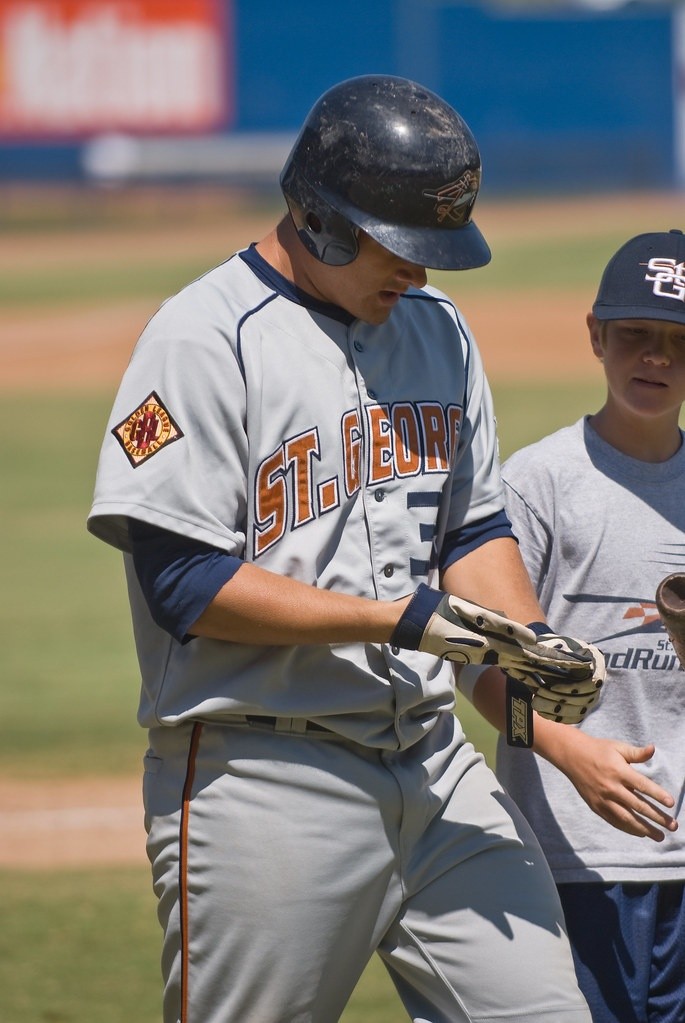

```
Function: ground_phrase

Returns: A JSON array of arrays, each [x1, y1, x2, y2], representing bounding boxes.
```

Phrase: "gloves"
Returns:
[[500, 621, 606, 747], [389, 582, 592, 689]]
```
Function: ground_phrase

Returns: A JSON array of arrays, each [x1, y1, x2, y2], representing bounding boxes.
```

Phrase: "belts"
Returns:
[[246, 712, 335, 733]]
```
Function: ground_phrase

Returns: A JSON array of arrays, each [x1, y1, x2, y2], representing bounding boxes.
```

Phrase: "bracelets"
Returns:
[[457, 662, 493, 702]]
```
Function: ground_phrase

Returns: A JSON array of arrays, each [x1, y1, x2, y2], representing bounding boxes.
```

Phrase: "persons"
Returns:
[[86, 73, 607, 1023], [449, 228, 685, 1023]]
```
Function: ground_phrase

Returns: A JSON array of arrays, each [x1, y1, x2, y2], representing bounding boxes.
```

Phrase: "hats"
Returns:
[[591, 229, 685, 325]]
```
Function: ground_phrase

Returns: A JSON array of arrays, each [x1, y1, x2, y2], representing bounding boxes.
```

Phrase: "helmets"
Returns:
[[279, 74, 493, 271]]
